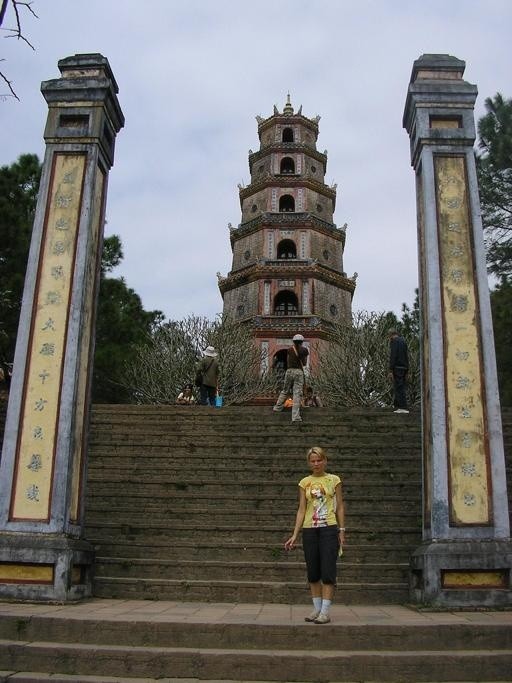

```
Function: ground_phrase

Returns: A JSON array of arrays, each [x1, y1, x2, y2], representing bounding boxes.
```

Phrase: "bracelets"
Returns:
[[338, 527, 346, 531]]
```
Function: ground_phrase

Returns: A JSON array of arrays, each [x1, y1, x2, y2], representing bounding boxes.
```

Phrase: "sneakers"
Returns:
[[304, 610, 330, 623], [395, 408, 408, 413]]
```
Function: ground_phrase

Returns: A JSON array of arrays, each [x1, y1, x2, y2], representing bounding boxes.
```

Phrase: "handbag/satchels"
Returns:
[[207, 397, 222, 406], [196, 374, 202, 386]]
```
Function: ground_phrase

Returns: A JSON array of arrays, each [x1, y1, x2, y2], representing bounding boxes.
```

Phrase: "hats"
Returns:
[[202, 346, 217, 358], [293, 334, 304, 341], [303, 366, 310, 384]]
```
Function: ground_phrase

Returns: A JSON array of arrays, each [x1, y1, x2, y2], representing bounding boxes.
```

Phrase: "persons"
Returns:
[[283, 392, 294, 408], [176, 384, 194, 406], [284, 446, 346, 624], [302, 386, 324, 408], [195, 346, 220, 407], [388, 327, 410, 414], [272, 334, 310, 423]]
